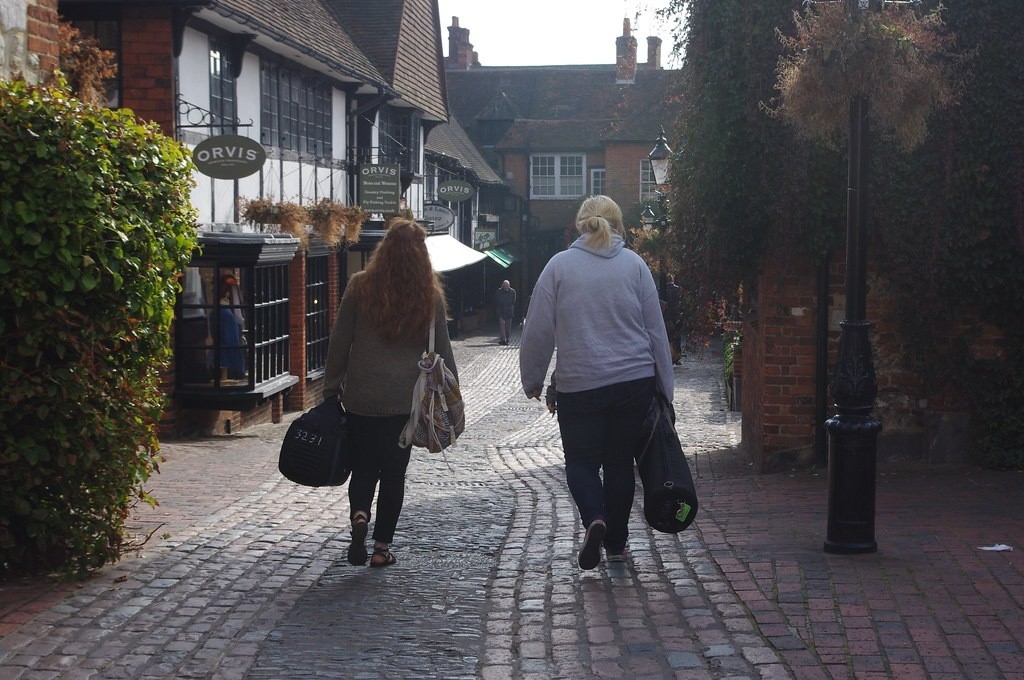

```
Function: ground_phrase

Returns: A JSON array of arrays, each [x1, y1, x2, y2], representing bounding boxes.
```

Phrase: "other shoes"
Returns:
[[607, 548, 626, 561], [501, 341, 506, 345], [578, 518, 607, 571]]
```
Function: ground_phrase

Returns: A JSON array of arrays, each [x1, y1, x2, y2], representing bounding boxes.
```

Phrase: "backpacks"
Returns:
[[398, 292, 465, 472]]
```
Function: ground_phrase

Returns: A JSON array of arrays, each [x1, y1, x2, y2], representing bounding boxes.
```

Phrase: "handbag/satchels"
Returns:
[[279, 402, 353, 487]]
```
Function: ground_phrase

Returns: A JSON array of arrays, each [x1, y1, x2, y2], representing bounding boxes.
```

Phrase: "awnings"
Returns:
[[424, 234, 487, 271], [483, 246, 518, 269]]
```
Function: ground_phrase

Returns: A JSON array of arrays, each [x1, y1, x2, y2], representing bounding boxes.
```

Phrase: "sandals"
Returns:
[[348, 514, 368, 565], [370, 544, 396, 567]]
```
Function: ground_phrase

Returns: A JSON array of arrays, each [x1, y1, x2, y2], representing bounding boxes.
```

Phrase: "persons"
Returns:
[[321, 216, 459, 569], [658, 273, 683, 354], [211, 275, 246, 373], [496, 279, 516, 345], [519, 195, 677, 570]]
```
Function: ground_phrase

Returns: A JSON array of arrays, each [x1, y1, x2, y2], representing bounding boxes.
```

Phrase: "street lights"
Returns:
[[648, 125, 675, 301]]
